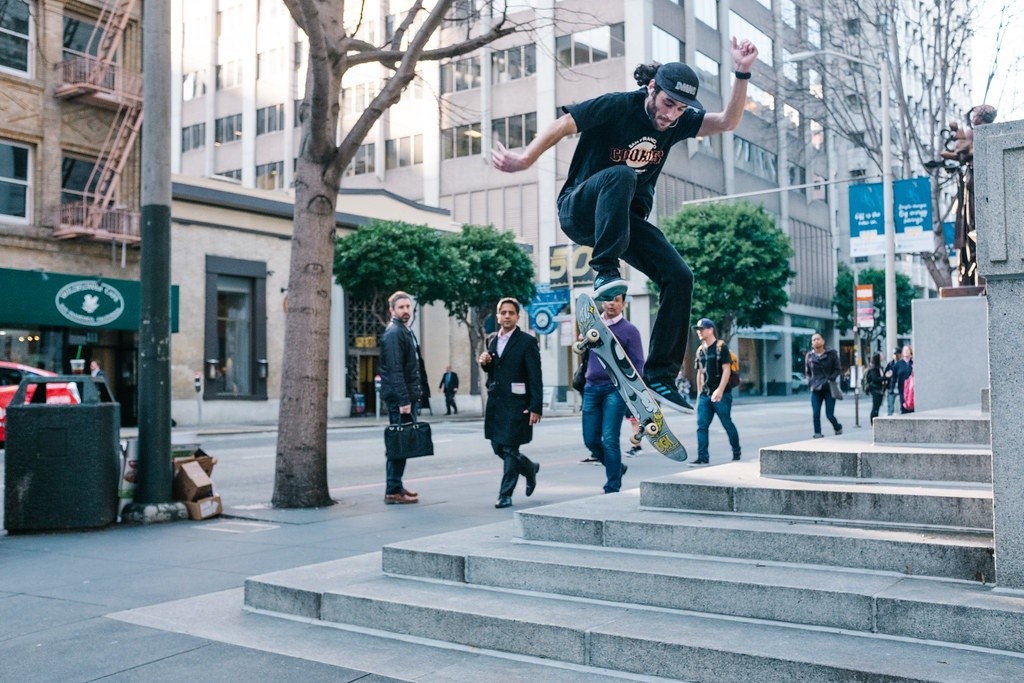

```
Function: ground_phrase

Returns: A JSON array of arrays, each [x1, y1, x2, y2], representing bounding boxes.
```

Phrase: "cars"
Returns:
[[0, 358, 82, 449]]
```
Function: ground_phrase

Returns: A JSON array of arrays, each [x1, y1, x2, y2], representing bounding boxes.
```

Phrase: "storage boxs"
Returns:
[[178, 462, 213, 501], [187, 494, 223, 519]]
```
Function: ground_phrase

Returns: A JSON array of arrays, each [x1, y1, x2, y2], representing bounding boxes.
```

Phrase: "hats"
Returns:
[[655, 62, 703, 110], [691, 317, 715, 330]]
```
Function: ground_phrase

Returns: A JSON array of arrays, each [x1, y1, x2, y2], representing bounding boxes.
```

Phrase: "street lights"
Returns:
[[786, 47, 899, 399]]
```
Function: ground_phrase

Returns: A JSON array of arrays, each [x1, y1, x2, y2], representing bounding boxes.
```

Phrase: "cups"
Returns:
[[70, 359, 85, 374]]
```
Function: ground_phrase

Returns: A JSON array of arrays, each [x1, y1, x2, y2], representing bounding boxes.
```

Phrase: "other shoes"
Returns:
[[733, 451, 741, 461], [454, 409, 457, 414], [401, 489, 418, 497], [445, 412, 451, 415], [618, 465, 628, 488], [385, 493, 417, 504], [624, 446, 642, 457], [577, 458, 602, 465], [687, 458, 709, 466], [525, 463, 540, 496], [813, 433, 823, 438], [495, 496, 512, 508], [834, 429, 842, 435]]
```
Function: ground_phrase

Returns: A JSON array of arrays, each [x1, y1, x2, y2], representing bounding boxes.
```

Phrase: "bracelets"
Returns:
[[735, 70, 752, 79]]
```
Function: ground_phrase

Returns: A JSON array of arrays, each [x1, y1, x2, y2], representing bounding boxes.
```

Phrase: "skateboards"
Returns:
[[571, 293, 688, 463]]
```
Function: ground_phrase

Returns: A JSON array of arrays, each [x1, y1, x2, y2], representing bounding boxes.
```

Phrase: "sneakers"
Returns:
[[643, 376, 694, 414], [592, 268, 628, 302]]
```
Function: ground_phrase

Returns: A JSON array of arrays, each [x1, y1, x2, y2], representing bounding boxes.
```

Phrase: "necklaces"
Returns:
[[643, 102, 679, 128]]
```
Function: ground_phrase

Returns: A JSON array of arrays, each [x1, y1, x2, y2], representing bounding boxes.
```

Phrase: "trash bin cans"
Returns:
[[3, 376, 122, 534]]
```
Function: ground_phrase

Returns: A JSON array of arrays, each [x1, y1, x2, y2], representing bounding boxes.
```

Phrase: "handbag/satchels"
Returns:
[[383, 412, 435, 460], [829, 381, 844, 400], [903, 372, 915, 410]]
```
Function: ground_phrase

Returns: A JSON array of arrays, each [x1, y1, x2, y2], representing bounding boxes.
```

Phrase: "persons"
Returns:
[[377, 291, 431, 505], [478, 297, 542, 510], [689, 317, 741, 468], [964, 103, 998, 266], [578, 292, 645, 497], [805, 334, 843, 438], [439, 366, 459, 415], [492, 36, 758, 415], [867, 345, 913, 425], [577, 349, 643, 465], [89, 359, 107, 402]]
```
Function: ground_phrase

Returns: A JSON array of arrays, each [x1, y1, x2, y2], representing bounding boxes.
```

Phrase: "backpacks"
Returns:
[[861, 367, 881, 395], [699, 340, 740, 387]]
